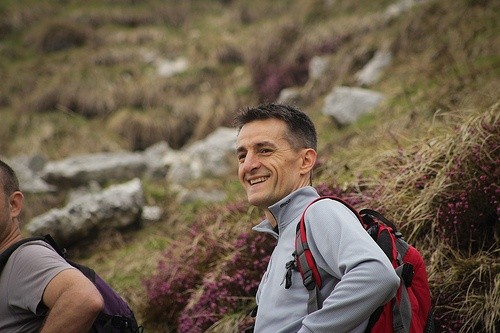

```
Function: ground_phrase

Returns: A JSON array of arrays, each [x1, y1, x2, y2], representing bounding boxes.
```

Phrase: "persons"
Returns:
[[1, 161, 106, 333], [234, 100, 403, 333]]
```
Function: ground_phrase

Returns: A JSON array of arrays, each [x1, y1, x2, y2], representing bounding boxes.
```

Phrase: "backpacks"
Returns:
[[0, 234, 143, 332], [281, 195, 434, 333]]
